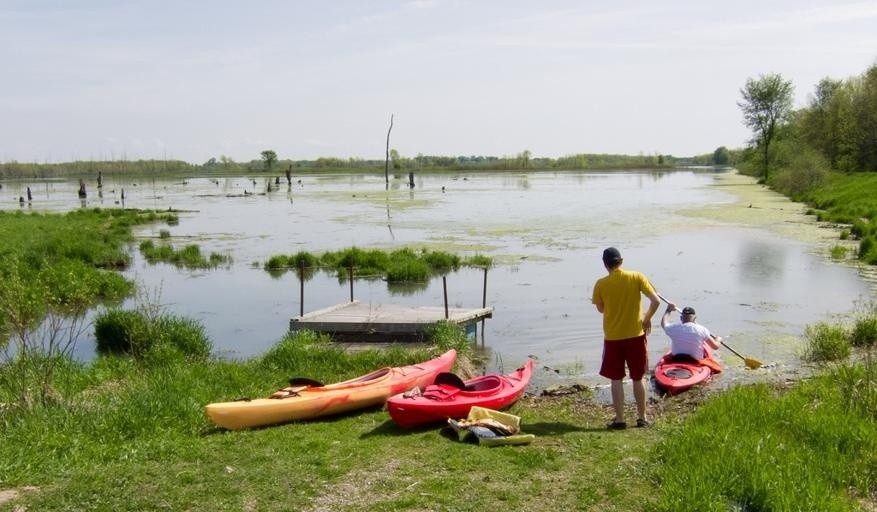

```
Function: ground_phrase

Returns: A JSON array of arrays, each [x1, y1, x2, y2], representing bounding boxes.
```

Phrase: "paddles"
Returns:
[[648, 283, 764, 370]]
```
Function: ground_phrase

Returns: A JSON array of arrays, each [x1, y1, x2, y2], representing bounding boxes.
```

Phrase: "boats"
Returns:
[[385, 360, 533, 430], [653, 342, 712, 394], [200, 347, 459, 433]]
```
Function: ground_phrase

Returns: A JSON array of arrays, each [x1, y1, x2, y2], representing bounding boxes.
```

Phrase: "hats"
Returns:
[[603, 247, 621, 261], [683, 307, 695, 315]]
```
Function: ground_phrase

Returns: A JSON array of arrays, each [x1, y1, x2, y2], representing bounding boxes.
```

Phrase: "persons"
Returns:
[[97, 171, 102, 188], [284, 163, 292, 183], [76, 178, 86, 197], [661, 303, 722, 365], [592, 247, 661, 430]]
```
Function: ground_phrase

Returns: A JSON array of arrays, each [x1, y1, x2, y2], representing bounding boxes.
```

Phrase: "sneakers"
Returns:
[[637, 419, 649, 428], [608, 421, 627, 429]]
